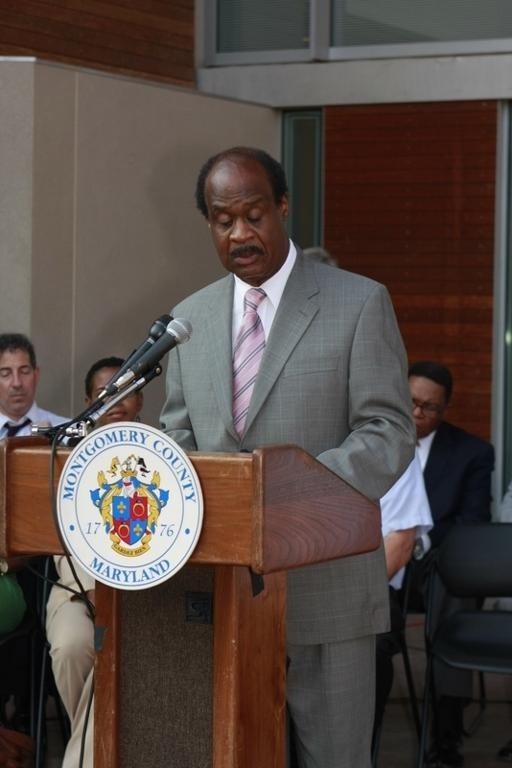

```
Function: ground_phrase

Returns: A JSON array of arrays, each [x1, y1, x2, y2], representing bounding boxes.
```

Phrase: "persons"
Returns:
[[370, 357, 499, 767], [155, 141, 419, 768], [42, 352, 147, 767], [0, 329, 84, 729], [370, 453, 433, 632]]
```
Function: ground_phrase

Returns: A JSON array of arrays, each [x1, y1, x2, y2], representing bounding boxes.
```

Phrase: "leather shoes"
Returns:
[[424, 742, 463, 768]]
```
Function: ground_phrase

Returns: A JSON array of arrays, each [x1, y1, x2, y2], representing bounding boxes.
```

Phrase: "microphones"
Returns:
[[108, 317, 193, 401], [97, 314, 174, 397]]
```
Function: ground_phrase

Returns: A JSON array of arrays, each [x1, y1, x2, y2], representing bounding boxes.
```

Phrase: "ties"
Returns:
[[4, 419, 31, 437], [232, 288, 267, 440]]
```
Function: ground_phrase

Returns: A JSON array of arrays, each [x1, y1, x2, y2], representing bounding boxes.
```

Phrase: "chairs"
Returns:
[[420, 524, 512, 768], [387, 593, 488, 744]]
